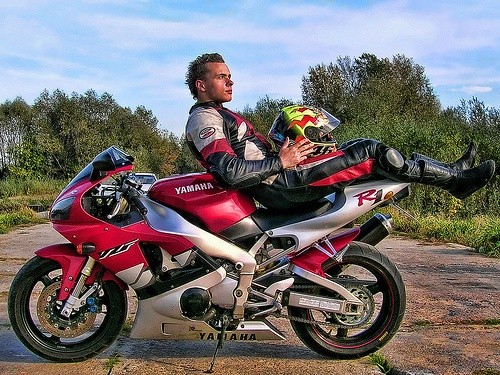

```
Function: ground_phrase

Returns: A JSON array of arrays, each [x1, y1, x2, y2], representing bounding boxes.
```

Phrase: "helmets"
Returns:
[[268, 104, 340, 157]]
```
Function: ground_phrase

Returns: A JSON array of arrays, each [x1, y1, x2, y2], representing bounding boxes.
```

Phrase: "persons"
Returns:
[[181, 52, 497, 213]]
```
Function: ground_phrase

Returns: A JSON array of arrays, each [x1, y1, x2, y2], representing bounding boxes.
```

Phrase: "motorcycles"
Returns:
[[7, 144, 412, 364]]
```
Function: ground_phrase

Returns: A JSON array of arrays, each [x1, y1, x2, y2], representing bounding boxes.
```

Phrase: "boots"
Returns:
[[417, 159, 494, 199], [411, 139, 478, 171]]
